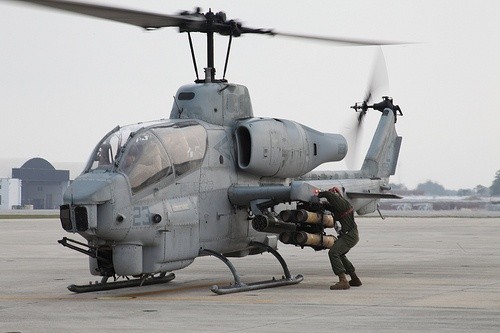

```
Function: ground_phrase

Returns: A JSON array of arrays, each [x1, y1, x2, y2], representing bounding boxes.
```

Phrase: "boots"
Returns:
[[349, 272, 363, 287], [330, 274, 350, 289]]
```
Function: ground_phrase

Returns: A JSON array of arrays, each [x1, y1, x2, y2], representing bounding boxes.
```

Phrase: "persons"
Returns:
[[317, 187, 362, 291]]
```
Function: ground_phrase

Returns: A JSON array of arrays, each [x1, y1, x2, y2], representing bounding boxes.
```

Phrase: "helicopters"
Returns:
[[5, 0, 424, 296]]
